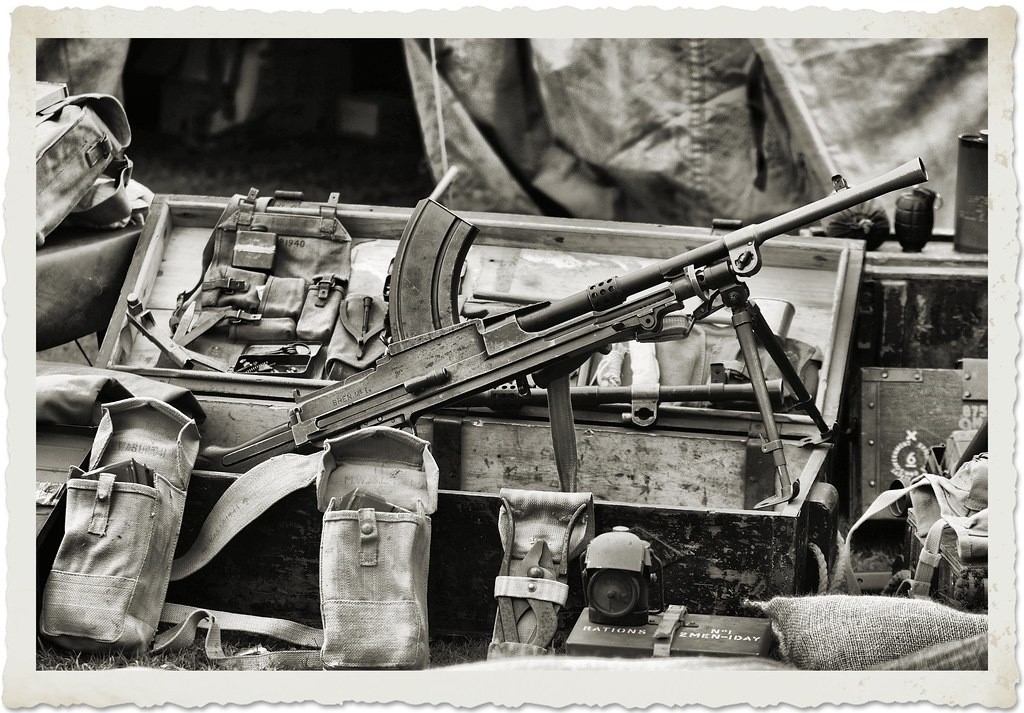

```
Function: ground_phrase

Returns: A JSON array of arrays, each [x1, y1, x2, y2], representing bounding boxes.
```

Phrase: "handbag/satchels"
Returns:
[[169, 187, 352, 383], [316, 426, 439, 670], [845, 452, 988, 600], [36, 93, 134, 248], [40, 397, 202, 659]]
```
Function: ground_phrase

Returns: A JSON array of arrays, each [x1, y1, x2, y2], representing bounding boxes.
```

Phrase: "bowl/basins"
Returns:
[[749, 298, 796, 344]]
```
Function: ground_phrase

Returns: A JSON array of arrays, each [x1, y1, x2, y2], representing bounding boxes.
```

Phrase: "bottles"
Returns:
[[953, 128, 988, 255]]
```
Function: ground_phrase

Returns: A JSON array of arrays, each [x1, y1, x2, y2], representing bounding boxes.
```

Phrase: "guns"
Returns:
[[203, 155, 930, 511]]
